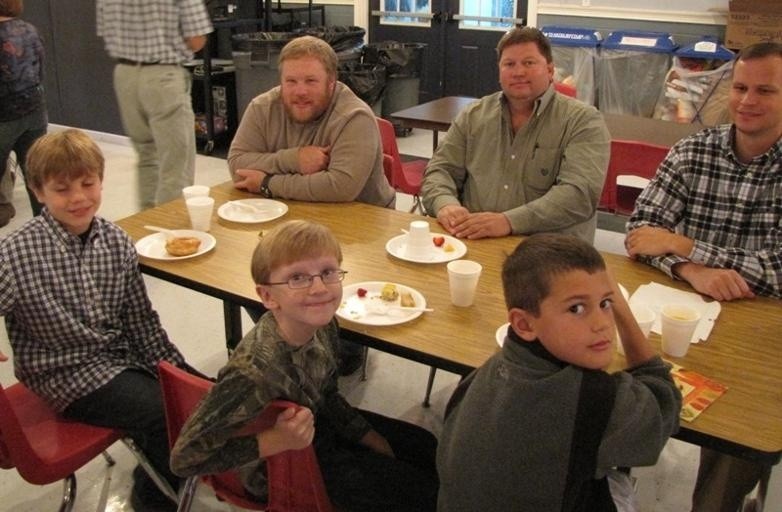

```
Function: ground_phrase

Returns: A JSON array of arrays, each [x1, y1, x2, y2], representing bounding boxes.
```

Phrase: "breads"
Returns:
[[165, 235, 201, 256]]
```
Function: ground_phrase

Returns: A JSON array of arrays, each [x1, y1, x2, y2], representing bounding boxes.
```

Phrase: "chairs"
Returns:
[[0, 376, 178, 505], [377, 155, 397, 191], [373, 114, 428, 214], [158, 361, 431, 512], [601, 139, 671, 223]]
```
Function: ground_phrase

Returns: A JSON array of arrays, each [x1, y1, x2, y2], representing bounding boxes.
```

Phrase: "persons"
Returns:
[[226, 35, 397, 209], [0, 128, 217, 512], [435, 232, 684, 512], [95, 0, 214, 209], [623, 42, 782, 303], [169, 219, 442, 512], [420, 26, 612, 246], [0, 0, 49, 227]]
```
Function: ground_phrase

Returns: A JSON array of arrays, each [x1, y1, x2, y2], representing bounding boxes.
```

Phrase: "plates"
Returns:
[[335, 281, 426, 327], [135, 229, 215, 261], [383, 232, 467, 264], [494, 323, 513, 349], [217, 199, 288, 224]]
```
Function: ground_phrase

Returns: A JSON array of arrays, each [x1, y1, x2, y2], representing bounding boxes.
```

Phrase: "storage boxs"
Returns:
[[724, 7, 781, 49]]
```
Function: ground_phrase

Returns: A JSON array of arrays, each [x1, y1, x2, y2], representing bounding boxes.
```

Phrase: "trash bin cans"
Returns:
[[363, 41, 426, 136], [230, 32, 317, 123], [337, 62, 387, 116], [292, 25, 366, 65]]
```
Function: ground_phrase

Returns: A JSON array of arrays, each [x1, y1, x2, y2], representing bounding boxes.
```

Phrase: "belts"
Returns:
[[117, 58, 159, 66]]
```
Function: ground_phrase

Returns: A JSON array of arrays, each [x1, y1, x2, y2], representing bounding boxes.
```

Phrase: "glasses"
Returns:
[[262, 267, 348, 289]]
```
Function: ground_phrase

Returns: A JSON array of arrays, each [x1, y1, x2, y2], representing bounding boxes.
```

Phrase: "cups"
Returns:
[[660, 304, 701, 358], [405, 221, 435, 258], [447, 260, 482, 308], [185, 196, 214, 233], [182, 185, 210, 200], [616, 304, 656, 358]]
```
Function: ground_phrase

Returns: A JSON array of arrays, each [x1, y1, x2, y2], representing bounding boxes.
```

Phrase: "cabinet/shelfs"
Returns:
[[189, 0, 236, 153]]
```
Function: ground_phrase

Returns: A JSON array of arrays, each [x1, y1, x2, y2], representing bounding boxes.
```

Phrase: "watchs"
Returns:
[[259, 173, 275, 199]]
